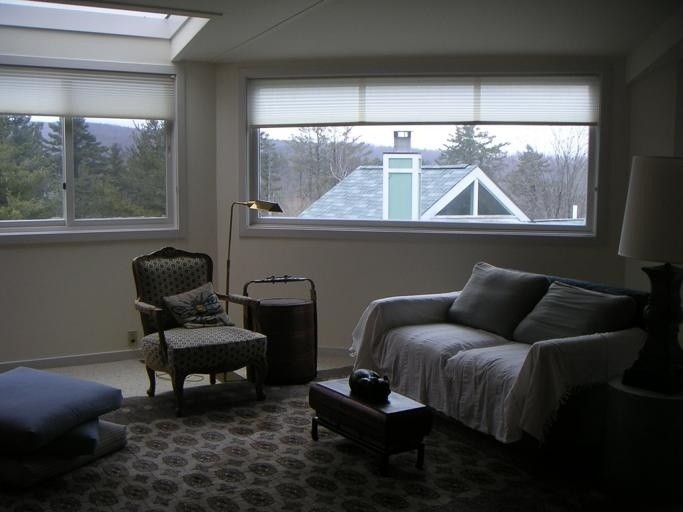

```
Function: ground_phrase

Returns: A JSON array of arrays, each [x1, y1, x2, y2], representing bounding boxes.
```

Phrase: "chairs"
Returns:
[[131, 248, 267, 417]]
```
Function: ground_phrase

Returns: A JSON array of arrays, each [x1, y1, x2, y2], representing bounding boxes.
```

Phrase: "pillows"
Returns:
[[1, 363, 129, 488], [448, 261, 638, 346], [162, 282, 234, 330]]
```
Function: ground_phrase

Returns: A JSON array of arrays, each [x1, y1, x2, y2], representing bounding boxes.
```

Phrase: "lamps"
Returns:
[[225, 199, 283, 315], [614, 155, 682, 395]]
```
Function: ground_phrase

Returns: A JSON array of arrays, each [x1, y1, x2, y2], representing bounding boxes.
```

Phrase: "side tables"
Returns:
[[604, 375, 682, 495]]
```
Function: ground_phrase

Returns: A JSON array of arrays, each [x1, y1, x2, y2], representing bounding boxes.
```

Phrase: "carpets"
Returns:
[[1, 366, 631, 511]]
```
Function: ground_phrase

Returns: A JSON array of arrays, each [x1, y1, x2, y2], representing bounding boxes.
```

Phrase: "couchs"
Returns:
[[366, 269, 650, 454]]
